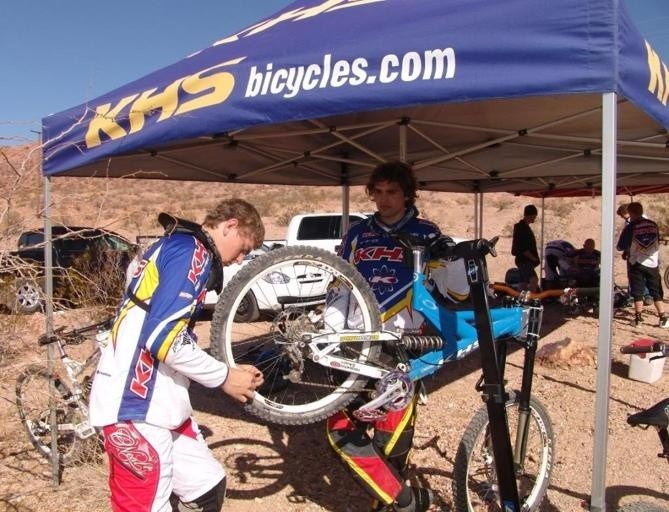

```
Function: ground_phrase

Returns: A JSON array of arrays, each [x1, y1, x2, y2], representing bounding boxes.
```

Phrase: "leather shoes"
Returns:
[[645, 298, 653, 305]]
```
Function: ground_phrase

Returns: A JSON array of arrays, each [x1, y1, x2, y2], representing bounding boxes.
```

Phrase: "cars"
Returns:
[[13, 226, 141, 279], [123, 234, 337, 326], [0, 253, 49, 314]]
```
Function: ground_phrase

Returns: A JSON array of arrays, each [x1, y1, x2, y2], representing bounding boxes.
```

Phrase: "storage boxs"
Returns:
[[627, 352, 667, 385]]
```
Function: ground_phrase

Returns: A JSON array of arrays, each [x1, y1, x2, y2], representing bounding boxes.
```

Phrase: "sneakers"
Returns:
[[659, 314, 669, 328], [630, 320, 642, 328], [383, 486, 433, 512]]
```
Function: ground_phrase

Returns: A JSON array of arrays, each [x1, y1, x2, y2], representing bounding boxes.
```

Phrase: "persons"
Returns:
[[616, 204, 649, 227], [545, 239, 575, 280], [511, 204, 540, 292], [88, 197, 266, 512], [616, 202, 668, 329], [565, 238, 601, 318], [322, 161, 472, 512]]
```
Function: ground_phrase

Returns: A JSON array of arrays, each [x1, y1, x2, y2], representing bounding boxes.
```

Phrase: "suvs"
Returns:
[[286, 213, 473, 261]]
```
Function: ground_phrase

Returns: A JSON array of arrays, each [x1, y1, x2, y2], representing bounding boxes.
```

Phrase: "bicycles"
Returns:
[[17, 309, 119, 469], [615, 343, 669, 464], [210, 234, 557, 507]]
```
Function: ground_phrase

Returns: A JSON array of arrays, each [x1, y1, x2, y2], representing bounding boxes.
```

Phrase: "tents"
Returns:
[[35, 2, 668, 512]]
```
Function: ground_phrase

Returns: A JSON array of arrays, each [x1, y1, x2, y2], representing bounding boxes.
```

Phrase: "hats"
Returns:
[[524, 205, 537, 215]]
